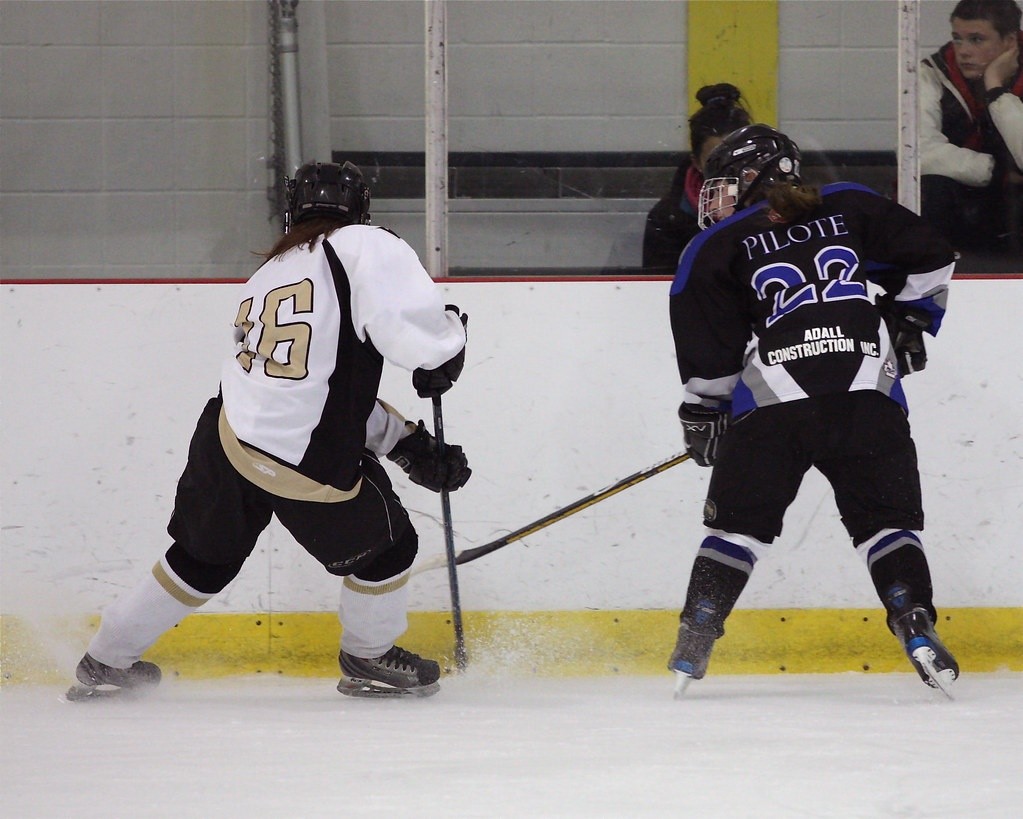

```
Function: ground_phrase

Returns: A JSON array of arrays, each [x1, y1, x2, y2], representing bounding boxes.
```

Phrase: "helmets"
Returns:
[[288, 159, 372, 227], [698, 123, 800, 231]]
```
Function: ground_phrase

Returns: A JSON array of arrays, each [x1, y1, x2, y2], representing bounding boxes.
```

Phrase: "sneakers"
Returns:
[[886, 586, 958, 699], [335, 645, 440, 699], [667, 595, 724, 697], [66, 654, 161, 702]]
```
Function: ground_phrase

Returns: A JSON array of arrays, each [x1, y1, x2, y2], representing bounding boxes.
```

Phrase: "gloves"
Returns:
[[412, 304, 468, 399], [678, 401, 729, 468], [874, 292, 927, 379], [386, 420, 471, 493]]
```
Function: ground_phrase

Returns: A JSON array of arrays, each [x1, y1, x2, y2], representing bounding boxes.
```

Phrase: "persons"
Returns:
[[920, 0, 1023, 274], [644, 83, 750, 268], [667, 124, 961, 701], [67, 160, 473, 703]]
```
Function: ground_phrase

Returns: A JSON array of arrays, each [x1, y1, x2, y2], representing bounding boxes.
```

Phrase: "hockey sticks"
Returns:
[[411, 336, 922, 578], [431, 395, 469, 676]]
